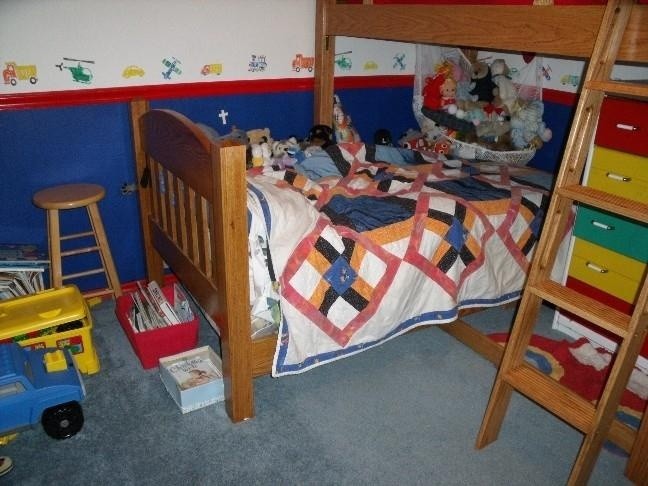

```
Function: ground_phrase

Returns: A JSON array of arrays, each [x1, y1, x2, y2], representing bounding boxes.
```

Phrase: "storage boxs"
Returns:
[[113, 281, 202, 371], [1, 284, 101, 378], [156, 341, 225, 415]]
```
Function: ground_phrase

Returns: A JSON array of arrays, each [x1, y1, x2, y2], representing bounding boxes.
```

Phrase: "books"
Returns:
[[126, 279, 222, 393], [0, 239, 50, 302]]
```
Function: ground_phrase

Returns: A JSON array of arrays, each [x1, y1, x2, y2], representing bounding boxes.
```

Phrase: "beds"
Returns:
[[132, 86, 578, 423], [311, 1, 647, 484]]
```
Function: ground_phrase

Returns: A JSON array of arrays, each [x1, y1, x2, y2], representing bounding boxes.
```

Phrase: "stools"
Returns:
[[33, 181, 121, 313]]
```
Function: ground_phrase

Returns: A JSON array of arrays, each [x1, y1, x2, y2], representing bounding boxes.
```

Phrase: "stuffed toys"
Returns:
[[421, 57, 554, 150], [332, 103, 362, 145], [190, 51, 554, 178], [216, 125, 337, 170], [374, 128, 429, 151]]
